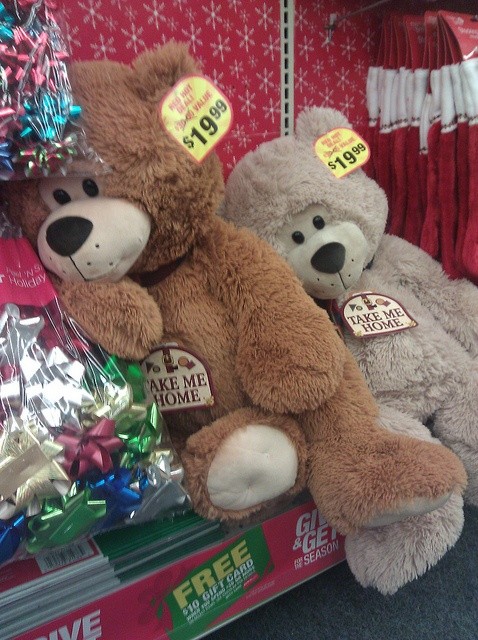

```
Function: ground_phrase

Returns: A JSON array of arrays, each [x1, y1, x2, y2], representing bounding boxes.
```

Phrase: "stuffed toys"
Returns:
[[216, 105, 477, 599], [6, 41, 468, 536]]
